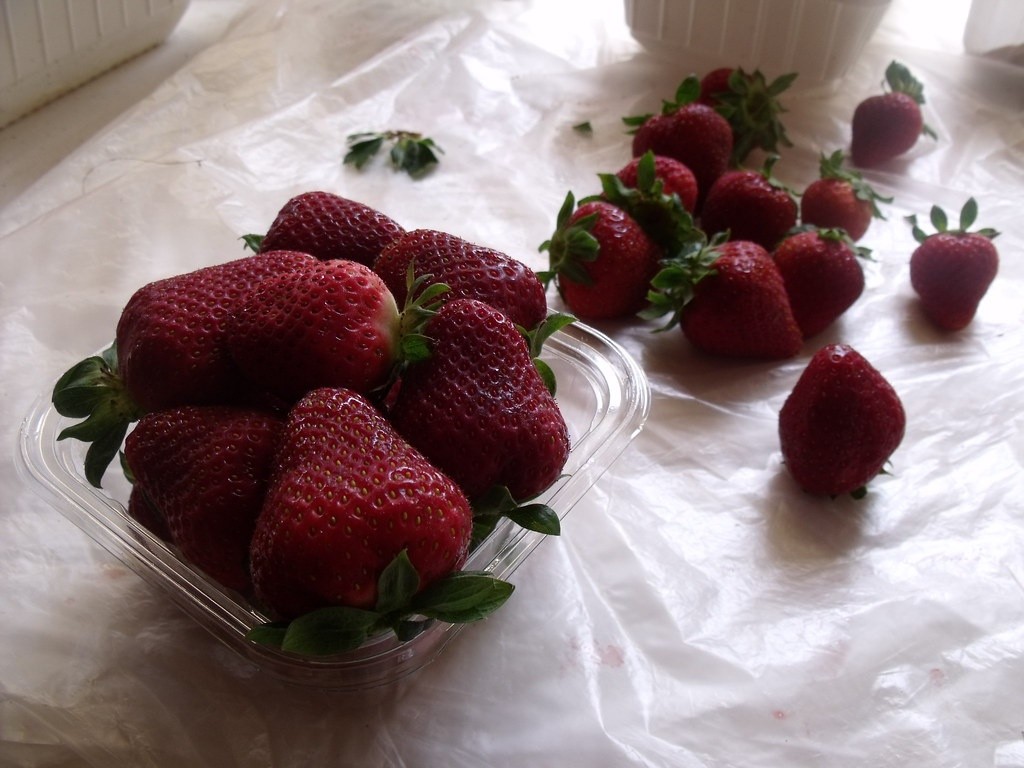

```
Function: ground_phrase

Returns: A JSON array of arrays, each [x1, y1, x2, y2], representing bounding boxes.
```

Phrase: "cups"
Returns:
[[625, 0, 896, 95]]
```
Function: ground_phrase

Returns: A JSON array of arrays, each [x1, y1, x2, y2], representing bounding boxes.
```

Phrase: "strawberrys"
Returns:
[[51, 191, 580, 685], [535, 62, 1001, 504]]
